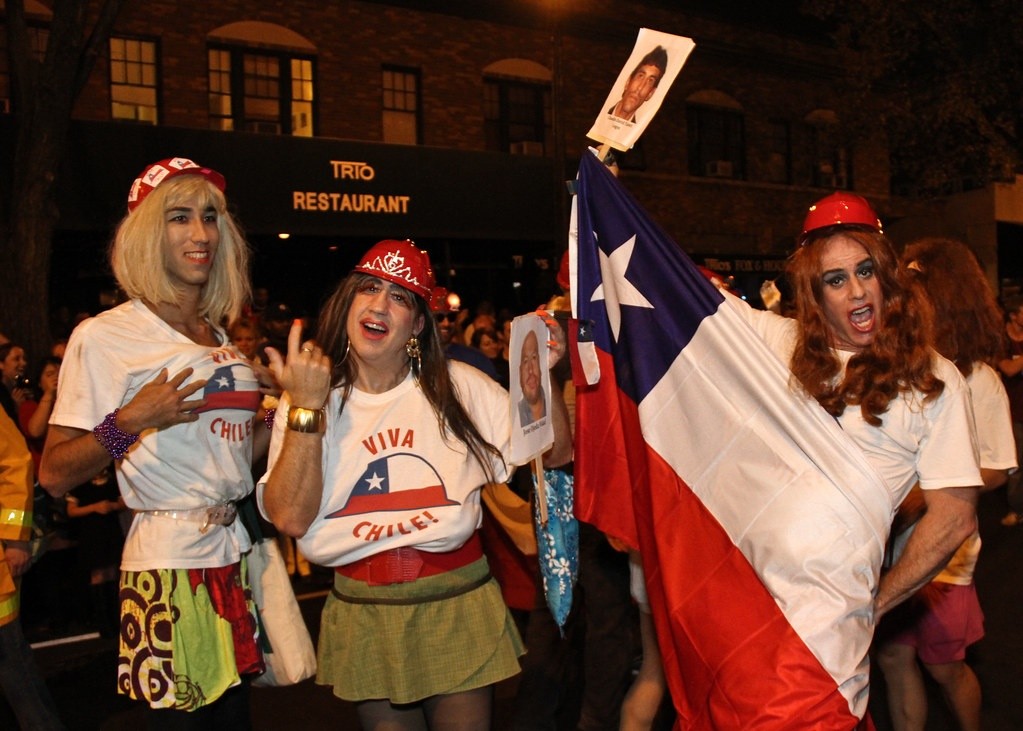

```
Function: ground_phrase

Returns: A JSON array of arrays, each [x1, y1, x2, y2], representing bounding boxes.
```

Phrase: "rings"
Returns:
[[301, 348, 312, 353]]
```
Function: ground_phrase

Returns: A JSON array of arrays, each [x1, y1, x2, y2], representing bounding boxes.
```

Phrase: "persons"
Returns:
[[587, 140, 985, 731], [258, 238, 573, 729], [517, 330, 547, 428], [607, 44, 668, 124], [40, 156, 273, 731], [2, 279, 1023, 696], [877, 237, 1019, 731], [1, 406, 63, 731], [621, 552, 664, 729]]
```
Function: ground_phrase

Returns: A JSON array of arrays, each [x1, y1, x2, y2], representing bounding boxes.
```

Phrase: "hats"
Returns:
[[427, 286, 452, 312], [801, 190, 884, 234], [127, 158, 228, 219], [351, 240, 437, 302]]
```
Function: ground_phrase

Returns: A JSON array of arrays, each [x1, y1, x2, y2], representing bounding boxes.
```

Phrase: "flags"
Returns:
[[568, 146, 898, 729]]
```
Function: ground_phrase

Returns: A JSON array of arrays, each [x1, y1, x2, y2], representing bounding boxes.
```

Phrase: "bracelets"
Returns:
[[286, 405, 326, 434], [263, 408, 276, 431], [93, 408, 139, 459]]
